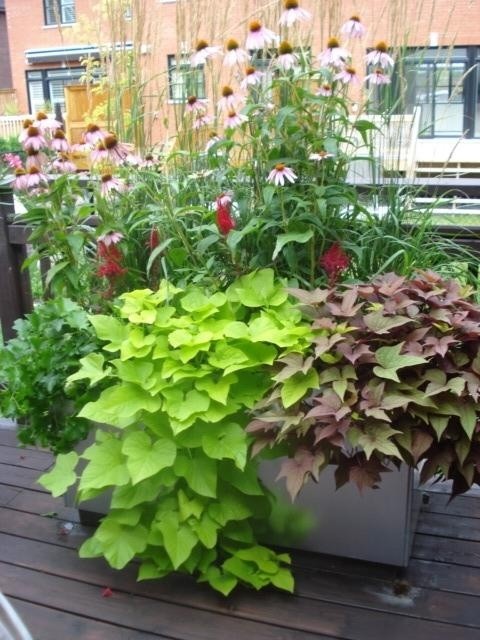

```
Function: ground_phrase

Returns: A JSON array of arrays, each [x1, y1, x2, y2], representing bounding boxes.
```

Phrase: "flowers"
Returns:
[[0, 0, 480, 597]]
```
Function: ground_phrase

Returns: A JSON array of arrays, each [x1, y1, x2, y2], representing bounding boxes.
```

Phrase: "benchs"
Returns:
[[370, 137, 480, 217]]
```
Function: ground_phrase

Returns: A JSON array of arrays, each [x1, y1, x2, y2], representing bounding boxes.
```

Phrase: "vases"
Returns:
[[54, 406, 425, 584]]
